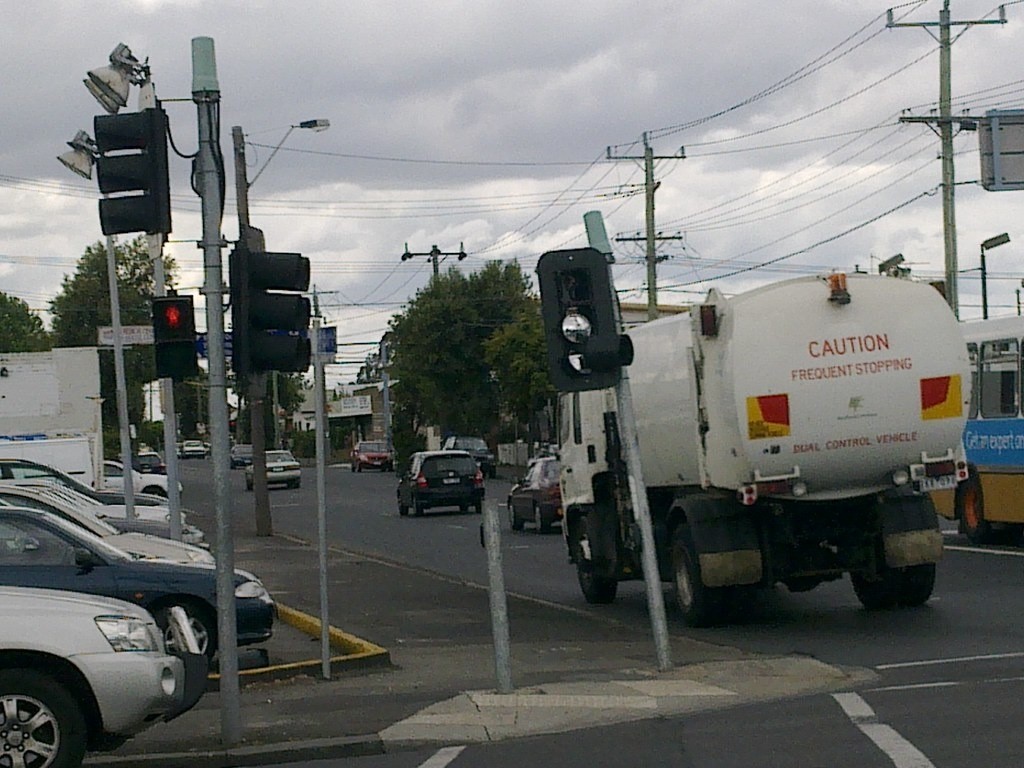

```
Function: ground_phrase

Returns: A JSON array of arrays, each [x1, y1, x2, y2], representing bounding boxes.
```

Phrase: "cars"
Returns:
[[181, 439, 208, 461], [1, 457, 218, 569], [0, 582, 208, 768], [103, 457, 183, 499], [138, 452, 166, 474], [440, 435, 497, 479], [243, 450, 301, 493], [0, 503, 277, 663], [230, 443, 256, 470], [397, 449, 487, 517], [508, 454, 562, 533], [348, 439, 396, 471]]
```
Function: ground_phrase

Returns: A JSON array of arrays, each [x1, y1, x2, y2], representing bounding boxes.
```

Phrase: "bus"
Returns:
[[928, 313, 1024, 546]]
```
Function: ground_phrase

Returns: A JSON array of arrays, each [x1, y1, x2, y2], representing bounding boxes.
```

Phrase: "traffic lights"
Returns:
[[150, 296, 197, 343], [536, 248, 623, 389], [93, 109, 172, 237], [228, 249, 310, 373]]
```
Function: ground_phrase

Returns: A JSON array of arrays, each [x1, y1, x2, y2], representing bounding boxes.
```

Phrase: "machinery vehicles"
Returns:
[[560, 272, 973, 628]]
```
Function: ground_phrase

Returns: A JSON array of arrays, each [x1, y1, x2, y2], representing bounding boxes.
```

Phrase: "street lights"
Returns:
[[232, 119, 332, 537], [979, 232, 1010, 320]]
